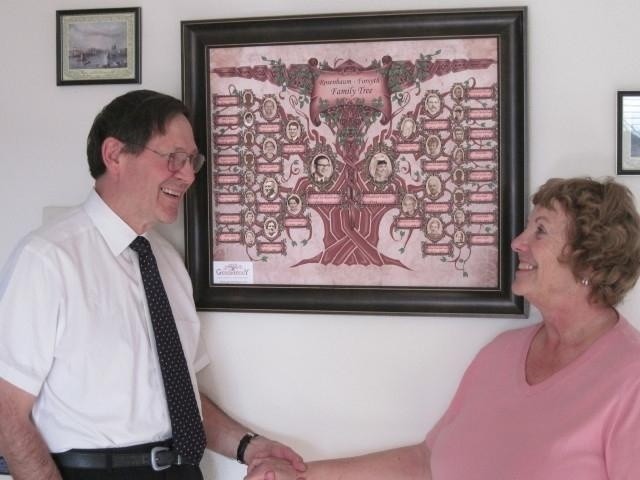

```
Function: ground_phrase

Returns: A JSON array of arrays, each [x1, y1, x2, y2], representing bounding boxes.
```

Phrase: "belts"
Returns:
[[56, 451, 184, 469]]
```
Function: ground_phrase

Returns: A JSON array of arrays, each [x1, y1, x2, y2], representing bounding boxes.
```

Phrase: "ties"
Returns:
[[128, 236, 207, 467]]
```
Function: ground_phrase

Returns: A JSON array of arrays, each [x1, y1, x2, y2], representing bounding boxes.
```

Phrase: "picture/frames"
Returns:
[[180, 4, 530, 321], [54, 6, 144, 87], [615, 90, 640, 176]]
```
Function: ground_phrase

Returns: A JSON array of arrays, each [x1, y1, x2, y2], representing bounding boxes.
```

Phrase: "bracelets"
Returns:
[[237, 431, 260, 465]]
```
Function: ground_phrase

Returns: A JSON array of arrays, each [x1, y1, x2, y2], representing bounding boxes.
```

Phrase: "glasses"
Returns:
[[122, 140, 206, 173]]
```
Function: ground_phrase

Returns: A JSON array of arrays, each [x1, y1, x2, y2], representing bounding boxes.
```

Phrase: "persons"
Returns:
[[244, 176, 640, 480], [0, 91, 306, 480], [244, 88, 465, 245]]
